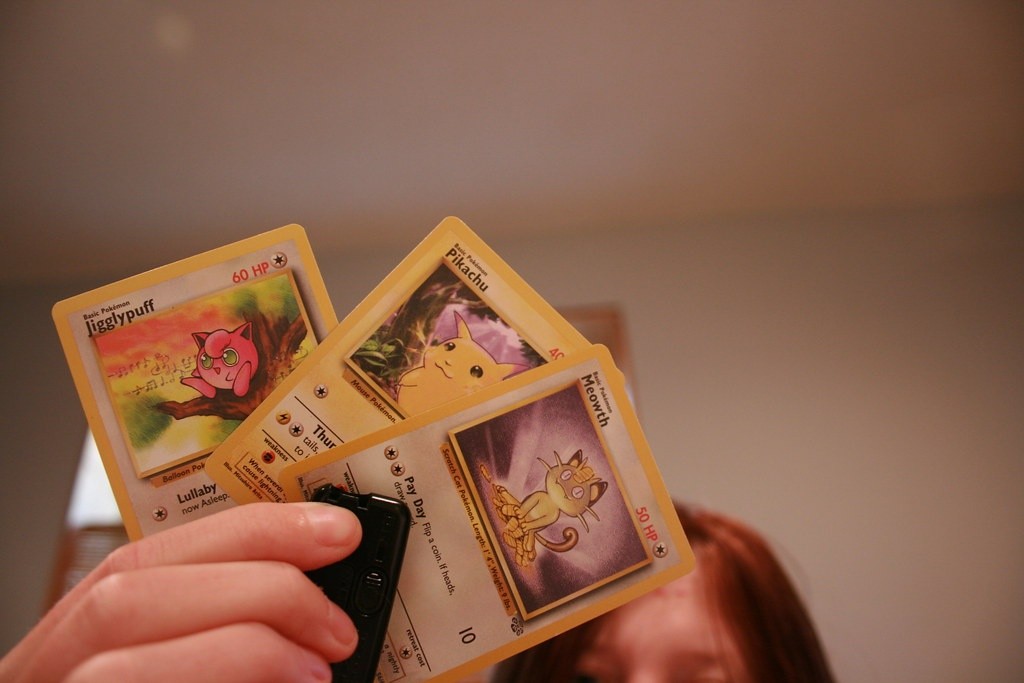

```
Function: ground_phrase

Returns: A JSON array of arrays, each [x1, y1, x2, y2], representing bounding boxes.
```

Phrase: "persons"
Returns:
[[0, 498, 836, 683]]
